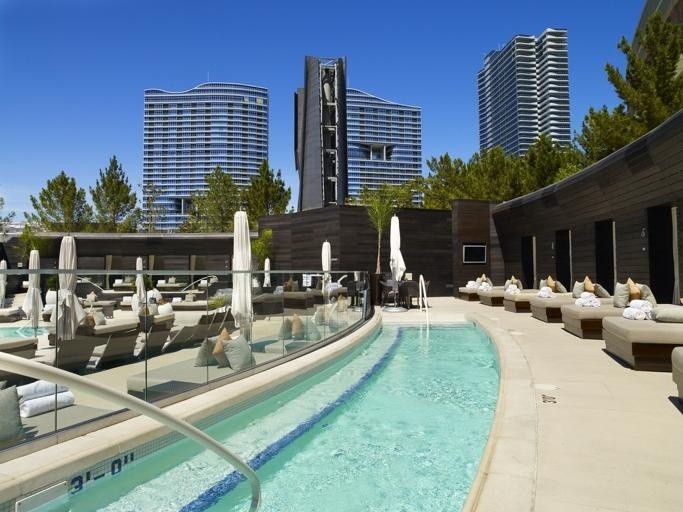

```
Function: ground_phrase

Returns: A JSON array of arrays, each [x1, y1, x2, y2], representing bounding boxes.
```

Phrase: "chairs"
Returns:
[[34, 309, 235, 376], [345, 270, 431, 310], [0, 282, 240, 322]]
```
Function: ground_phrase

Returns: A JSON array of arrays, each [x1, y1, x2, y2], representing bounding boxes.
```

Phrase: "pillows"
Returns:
[[0, 385, 26, 448], [279, 303, 352, 341], [192, 327, 256, 372], [475, 273, 657, 308]]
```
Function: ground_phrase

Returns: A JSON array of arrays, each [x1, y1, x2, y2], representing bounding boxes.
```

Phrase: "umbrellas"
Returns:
[[21, 247, 44, 340], [135, 256, 145, 305], [50, 234, 87, 341], [230, 206, 253, 340], [0, 258, 7, 309], [321, 239, 332, 283], [389, 213, 407, 307], [263, 256, 272, 287]]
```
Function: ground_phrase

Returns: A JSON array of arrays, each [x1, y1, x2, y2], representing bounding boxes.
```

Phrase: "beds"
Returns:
[[458, 287, 683, 372], [251, 283, 346, 314], [127, 348, 282, 402], [20, 400, 122, 447], [250, 324, 333, 356]]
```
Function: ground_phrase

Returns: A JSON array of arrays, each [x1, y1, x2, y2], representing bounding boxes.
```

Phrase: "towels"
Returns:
[[466, 280, 479, 289]]
